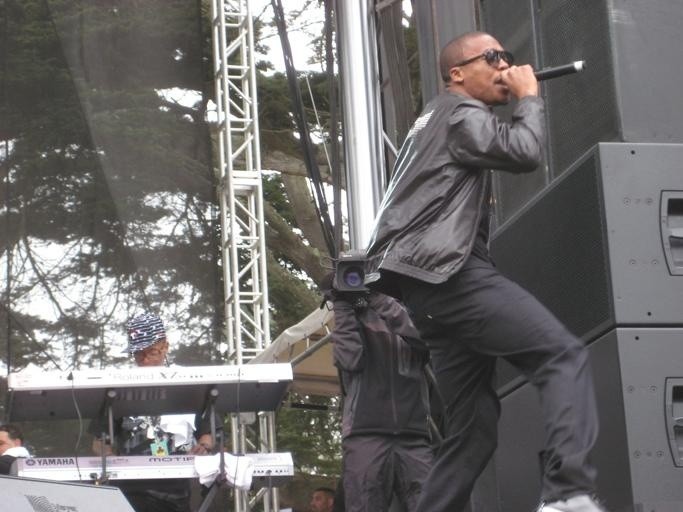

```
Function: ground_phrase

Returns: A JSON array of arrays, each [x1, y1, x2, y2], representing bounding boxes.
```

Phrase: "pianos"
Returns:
[[8, 450, 295, 488], [7, 362, 294, 423]]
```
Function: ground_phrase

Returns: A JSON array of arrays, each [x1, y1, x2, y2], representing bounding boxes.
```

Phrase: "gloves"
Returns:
[[319, 273, 354, 301]]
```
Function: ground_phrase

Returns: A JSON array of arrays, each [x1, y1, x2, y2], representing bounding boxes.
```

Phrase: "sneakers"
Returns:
[[536, 494, 609, 511]]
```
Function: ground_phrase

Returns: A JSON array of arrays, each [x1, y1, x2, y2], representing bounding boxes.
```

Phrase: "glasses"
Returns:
[[456, 49, 513, 66], [133, 342, 165, 361]]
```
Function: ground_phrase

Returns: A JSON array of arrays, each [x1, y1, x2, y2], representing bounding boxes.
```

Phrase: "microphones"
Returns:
[[534, 60, 586, 82]]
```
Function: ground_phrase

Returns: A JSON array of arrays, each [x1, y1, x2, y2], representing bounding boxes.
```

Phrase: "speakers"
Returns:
[[489, 141, 683, 398], [469, 328, 683, 512]]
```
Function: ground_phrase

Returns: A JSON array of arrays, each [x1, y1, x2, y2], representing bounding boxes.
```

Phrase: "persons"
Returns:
[[86, 314, 224, 512], [310, 487, 337, 512], [331, 254, 437, 512], [362, 31, 610, 512], [0, 424, 31, 475]]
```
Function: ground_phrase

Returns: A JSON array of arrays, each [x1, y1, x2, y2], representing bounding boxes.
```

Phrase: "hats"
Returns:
[[121, 315, 165, 353]]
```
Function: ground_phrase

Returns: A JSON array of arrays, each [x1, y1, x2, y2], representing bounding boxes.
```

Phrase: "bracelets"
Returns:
[[201, 443, 212, 454]]
